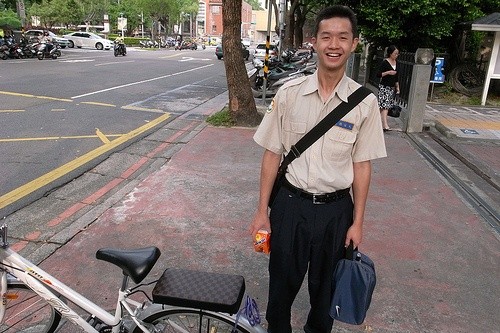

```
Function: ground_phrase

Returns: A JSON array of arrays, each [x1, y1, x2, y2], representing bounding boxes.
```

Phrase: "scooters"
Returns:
[[114, 39, 127, 56], [247, 47, 318, 98], [0, 35, 61, 60], [139, 36, 176, 48]]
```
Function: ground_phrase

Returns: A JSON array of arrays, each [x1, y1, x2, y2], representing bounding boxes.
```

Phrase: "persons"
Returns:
[[114, 33, 125, 55], [41, 32, 53, 55], [378, 45, 401, 131], [249, 6, 387, 333], [176, 35, 183, 47]]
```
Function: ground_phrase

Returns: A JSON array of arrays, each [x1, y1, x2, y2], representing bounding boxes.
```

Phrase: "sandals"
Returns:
[[383, 128, 392, 131]]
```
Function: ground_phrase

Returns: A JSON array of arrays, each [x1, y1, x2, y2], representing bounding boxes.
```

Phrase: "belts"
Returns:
[[284, 176, 350, 205]]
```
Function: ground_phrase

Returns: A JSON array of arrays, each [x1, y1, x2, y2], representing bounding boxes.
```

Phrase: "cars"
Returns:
[[22, 30, 71, 49], [174, 41, 197, 50], [62, 32, 114, 50], [254, 42, 279, 61], [215, 38, 250, 61]]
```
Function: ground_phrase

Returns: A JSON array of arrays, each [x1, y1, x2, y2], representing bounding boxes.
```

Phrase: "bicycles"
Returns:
[[0, 217, 266, 333]]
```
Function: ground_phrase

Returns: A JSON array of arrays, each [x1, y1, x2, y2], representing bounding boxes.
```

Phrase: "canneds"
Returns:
[[253, 229, 270, 253]]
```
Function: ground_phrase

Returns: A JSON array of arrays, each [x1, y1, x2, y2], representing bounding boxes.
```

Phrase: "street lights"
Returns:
[[185, 13, 192, 38]]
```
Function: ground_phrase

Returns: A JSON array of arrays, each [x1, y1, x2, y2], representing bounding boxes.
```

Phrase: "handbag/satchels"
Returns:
[[388, 94, 402, 117], [328, 241, 376, 326]]
